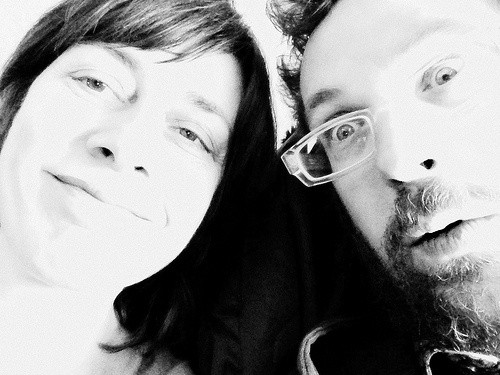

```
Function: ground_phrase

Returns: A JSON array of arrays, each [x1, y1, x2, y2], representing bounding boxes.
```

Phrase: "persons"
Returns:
[[0, 0, 273, 375], [274, 0, 500, 375]]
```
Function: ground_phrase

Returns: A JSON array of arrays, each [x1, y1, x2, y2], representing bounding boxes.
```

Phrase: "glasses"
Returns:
[[277, 42, 500, 188]]
